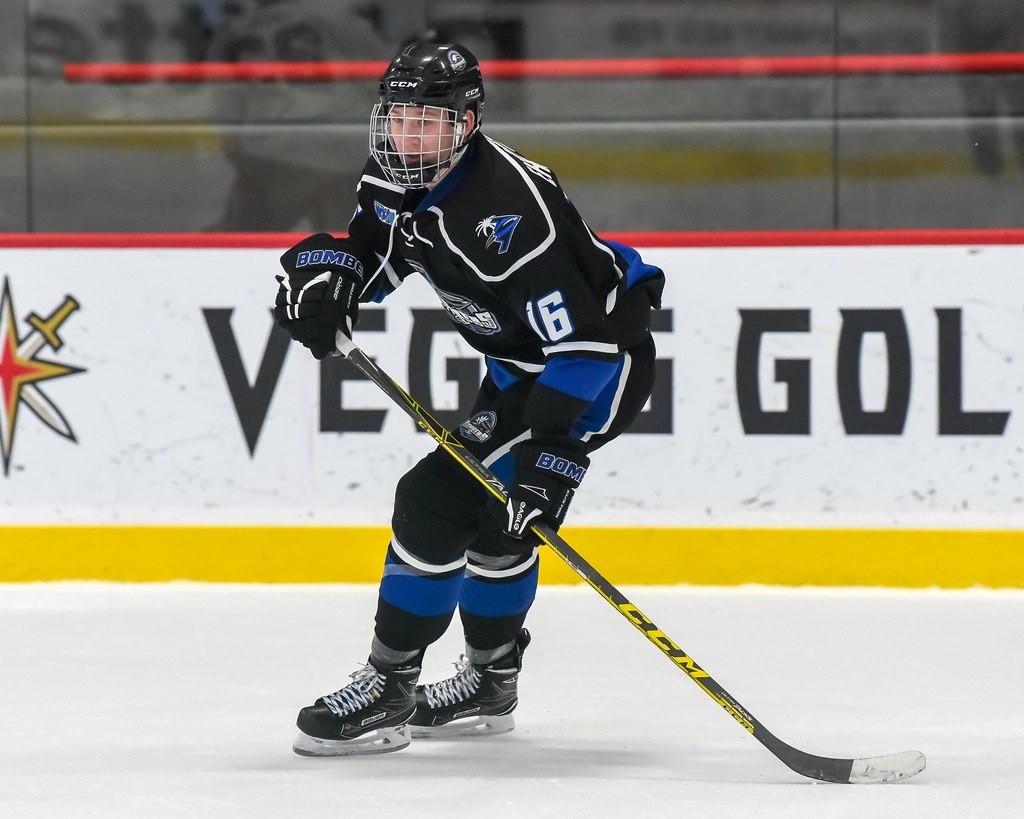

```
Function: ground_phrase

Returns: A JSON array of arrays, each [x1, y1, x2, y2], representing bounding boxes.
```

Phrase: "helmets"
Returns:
[[380, 38, 486, 109]]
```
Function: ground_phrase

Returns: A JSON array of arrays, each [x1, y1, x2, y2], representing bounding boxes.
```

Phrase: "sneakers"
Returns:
[[295, 655, 417, 756], [412, 627, 530, 741]]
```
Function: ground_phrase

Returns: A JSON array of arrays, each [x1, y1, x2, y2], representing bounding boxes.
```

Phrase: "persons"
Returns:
[[273, 40, 665, 756]]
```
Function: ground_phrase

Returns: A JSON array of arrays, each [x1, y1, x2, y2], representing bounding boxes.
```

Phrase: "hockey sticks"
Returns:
[[335, 327, 927, 783]]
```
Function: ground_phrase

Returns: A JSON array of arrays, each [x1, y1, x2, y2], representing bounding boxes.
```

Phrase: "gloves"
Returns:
[[502, 468, 575, 557], [274, 269, 358, 359]]
